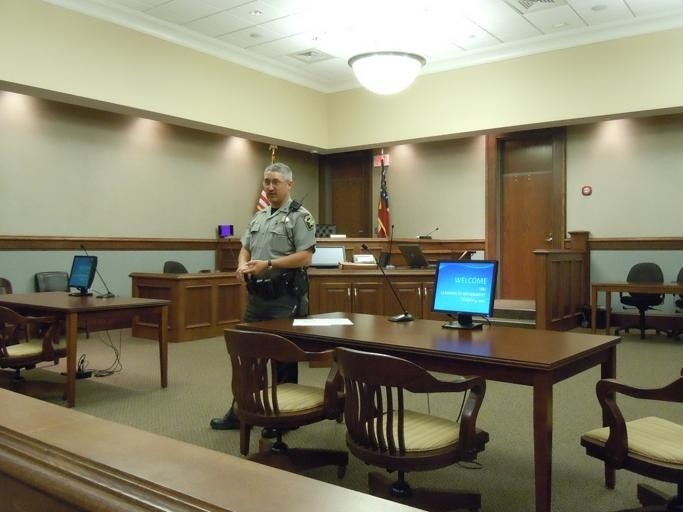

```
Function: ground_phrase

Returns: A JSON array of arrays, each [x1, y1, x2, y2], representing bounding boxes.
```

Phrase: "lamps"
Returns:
[[344, 46, 426, 99]]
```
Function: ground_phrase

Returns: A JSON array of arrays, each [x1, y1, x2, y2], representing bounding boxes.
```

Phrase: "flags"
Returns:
[[254, 158, 272, 211], [374, 158, 391, 239]]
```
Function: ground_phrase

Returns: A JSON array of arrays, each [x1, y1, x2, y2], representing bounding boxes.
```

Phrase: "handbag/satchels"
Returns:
[[245, 271, 306, 299]]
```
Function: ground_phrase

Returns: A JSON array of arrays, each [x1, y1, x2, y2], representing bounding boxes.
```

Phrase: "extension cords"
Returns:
[[75, 369, 92, 379]]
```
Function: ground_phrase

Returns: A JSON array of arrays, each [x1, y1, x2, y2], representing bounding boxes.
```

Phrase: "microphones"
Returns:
[[418, 226, 441, 239], [386, 224, 396, 266], [78, 243, 114, 299], [360, 242, 413, 322]]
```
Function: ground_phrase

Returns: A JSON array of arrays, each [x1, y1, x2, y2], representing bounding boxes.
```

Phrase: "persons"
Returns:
[[208, 162, 317, 440]]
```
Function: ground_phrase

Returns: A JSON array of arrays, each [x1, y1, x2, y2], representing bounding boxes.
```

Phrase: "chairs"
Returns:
[[222, 329, 349, 482], [164, 261, 189, 274], [666, 265, 683, 338], [35, 271, 90, 341], [335, 347, 490, 512], [615, 262, 666, 339], [1, 304, 68, 402], [0, 278, 32, 343], [579, 368, 683, 510]]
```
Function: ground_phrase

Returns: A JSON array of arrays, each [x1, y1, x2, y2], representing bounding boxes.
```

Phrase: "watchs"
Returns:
[[267, 259, 271, 271]]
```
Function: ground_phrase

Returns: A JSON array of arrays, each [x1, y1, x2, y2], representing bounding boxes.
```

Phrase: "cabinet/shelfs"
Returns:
[[387, 272, 459, 322], [311, 272, 388, 317]]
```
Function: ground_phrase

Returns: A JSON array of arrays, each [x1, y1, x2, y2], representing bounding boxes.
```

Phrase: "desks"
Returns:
[[591, 283, 683, 334], [235, 311, 622, 511], [127, 267, 246, 344], [0, 291, 176, 408]]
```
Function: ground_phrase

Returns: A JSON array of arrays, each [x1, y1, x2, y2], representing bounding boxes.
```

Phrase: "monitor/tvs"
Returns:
[[352, 254, 375, 264], [330, 233, 347, 239], [310, 246, 346, 268], [398, 246, 428, 267], [430, 260, 499, 330], [67, 256, 97, 297]]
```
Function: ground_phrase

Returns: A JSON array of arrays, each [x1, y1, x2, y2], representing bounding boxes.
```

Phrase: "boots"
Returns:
[[261, 422, 300, 438], [210, 401, 255, 430]]
